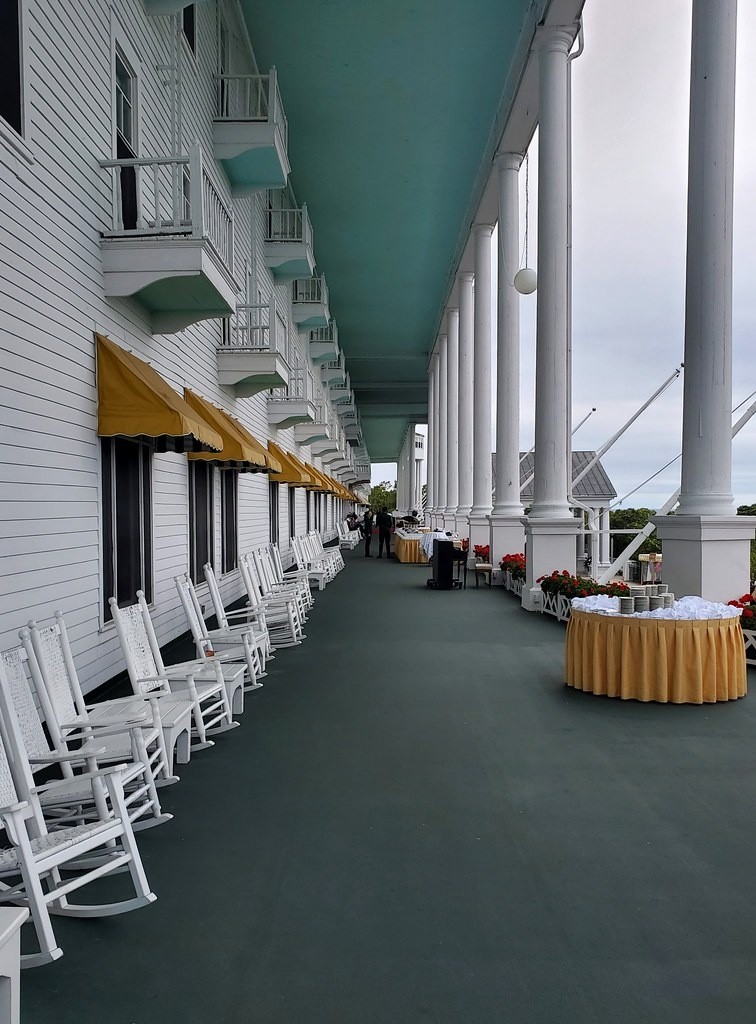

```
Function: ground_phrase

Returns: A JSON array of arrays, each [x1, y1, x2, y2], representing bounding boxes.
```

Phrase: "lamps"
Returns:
[[513, 152, 537, 296]]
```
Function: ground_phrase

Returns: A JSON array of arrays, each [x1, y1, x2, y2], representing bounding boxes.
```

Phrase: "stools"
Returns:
[[475, 563, 492, 589]]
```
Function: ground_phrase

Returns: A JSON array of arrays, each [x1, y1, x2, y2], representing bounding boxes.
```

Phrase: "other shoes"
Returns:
[[387, 556, 392, 558], [365, 554, 374, 557], [377, 556, 382, 558]]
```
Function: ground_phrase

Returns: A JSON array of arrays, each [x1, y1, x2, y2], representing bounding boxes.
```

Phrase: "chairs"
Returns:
[[336, 519, 363, 550], [0, 590, 242, 970], [173, 529, 345, 694]]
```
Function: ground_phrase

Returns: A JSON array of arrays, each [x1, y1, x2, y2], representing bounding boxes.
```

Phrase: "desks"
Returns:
[[565, 607, 748, 704], [394, 534, 427, 563], [0, 907, 30, 1024], [160, 664, 249, 727], [82, 700, 196, 775]]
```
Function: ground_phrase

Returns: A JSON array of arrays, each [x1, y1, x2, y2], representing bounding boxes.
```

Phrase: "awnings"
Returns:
[[93, 332, 371, 507]]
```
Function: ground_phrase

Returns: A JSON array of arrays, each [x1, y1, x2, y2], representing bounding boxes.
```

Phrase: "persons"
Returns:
[[376, 507, 394, 558], [348, 510, 375, 558]]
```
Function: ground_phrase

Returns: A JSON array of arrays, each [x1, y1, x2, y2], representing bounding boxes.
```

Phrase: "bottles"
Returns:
[[619, 584, 674, 614]]
